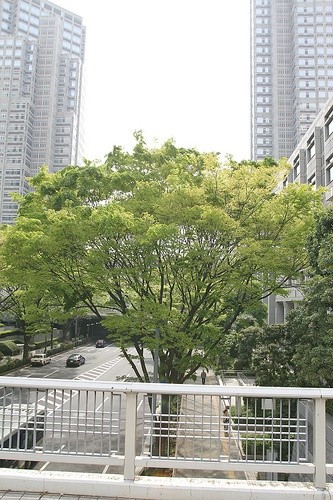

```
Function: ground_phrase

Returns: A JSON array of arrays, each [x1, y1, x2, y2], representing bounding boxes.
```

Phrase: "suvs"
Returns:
[[30, 353, 52, 366]]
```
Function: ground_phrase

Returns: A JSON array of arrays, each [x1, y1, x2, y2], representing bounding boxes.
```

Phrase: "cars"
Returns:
[[66, 353, 86, 367], [95, 339, 107, 348]]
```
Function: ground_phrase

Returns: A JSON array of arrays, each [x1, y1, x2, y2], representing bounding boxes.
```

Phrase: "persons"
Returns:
[[201, 371, 207, 385]]
